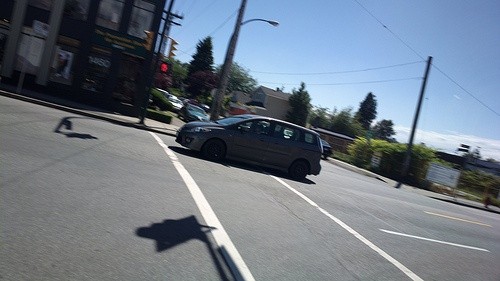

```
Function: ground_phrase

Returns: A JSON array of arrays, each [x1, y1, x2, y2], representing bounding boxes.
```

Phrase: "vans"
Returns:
[[175, 114, 324, 183]]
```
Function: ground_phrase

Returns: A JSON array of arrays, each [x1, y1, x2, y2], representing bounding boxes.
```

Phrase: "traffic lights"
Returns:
[[169, 38, 177, 57], [143, 28, 153, 51], [159, 61, 172, 74]]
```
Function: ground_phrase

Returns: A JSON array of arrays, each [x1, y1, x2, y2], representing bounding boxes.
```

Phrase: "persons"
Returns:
[[253, 123, 263, 135], [56, 53, 71, 73]]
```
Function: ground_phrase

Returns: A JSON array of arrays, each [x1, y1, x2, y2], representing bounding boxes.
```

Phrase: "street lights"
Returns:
[[213, 18, 279, 118]]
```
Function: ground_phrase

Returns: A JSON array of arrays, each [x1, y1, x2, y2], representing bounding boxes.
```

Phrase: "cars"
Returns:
[[320, 139, 332, 159], [179, 104, 212, 125], [155, 87, 200, 114]]
